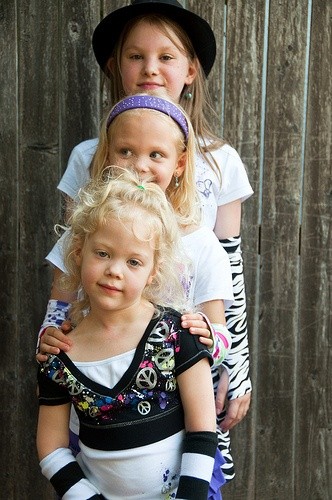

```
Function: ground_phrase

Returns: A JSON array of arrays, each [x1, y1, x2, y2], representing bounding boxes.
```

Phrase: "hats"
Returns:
[[91, 0, 217, 78]]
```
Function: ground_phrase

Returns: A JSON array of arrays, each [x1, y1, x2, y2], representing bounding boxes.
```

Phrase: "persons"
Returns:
[[37, 165, 217, 500], [37, 94, 231, 500], [58, 0, 252, 483]]
